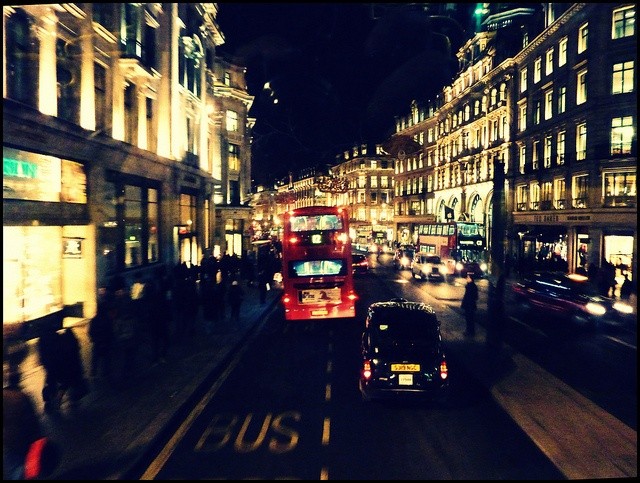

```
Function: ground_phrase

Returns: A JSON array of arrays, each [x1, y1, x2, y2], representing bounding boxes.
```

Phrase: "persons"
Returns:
[[587, 257, 634, 297], [460, 274, 478, 336], [3, 372, 43, 480], [38, 235, 283, 414]]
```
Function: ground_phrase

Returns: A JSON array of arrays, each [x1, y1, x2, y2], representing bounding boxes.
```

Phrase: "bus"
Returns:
[[282, 205, 356, 321], [417, 221, 487, 279]]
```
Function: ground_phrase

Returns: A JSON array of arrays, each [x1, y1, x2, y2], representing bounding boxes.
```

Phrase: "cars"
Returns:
[[511, 271, 631, 329], [394, 245, 415, 268], [352, 254, 368, 272], [359, 297, 448, 401]]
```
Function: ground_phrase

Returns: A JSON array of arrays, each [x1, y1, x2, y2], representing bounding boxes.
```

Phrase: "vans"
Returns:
[[410, 252, 442, 280]]
